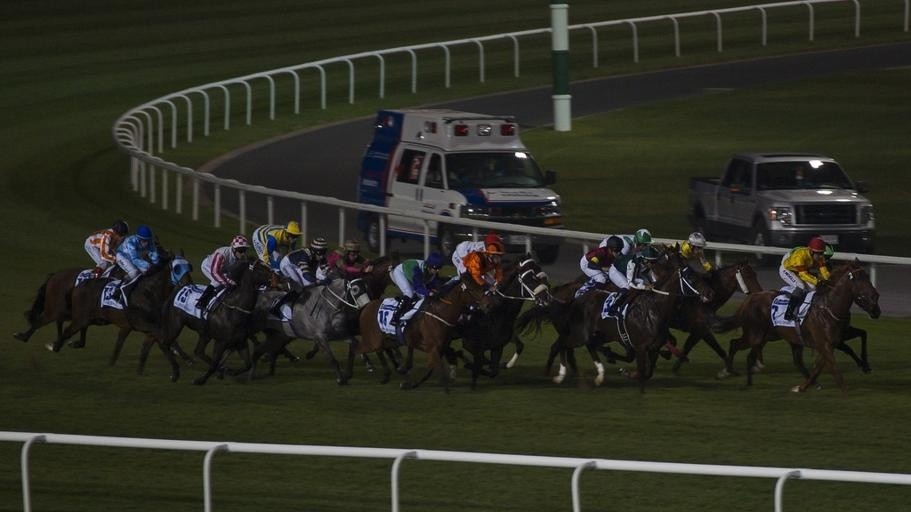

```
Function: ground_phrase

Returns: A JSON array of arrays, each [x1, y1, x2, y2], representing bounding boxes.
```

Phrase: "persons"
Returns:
[[608, 247, 661, 319], [575, 235, 625, 299], [666, 231, 714, 273], [792, 165, 814, 188], [252, 220, 305, 273], [114, 225, 155, 280], [600, 228, 653, 256], [779, 238, 832, 322], [328, 238, 375, 274], [200, 233, 252, 289], [451, 234, 505, 281], [269, 236, 333, 319], [408, 156, 506, 183], [388, 252, 446, 323], [450, 242, 507, 294], [83, 219, 130, 278], [781, 242, 835, 278]]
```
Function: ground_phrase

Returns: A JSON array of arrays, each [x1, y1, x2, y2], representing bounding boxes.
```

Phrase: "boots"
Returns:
[[608, 290, 630, 316], [94, 267, 104, 280], [574, 279, 598, 300], [113, 278, 130, 301], [195, 284, 215, 310], [784, 287, 807, 322], [270, 290, 299, 319], [390, 296, 412, 327]]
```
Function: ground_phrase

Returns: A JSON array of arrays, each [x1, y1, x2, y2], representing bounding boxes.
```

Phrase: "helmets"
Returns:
[[688, 231, 706, 247], [486, 234, 504, 245], [135, 224, 153, 240], [112, 219, 128, 233], [231, 235, 250, 249], [284, 221, 304, 235], [427, 252, 443, 267], [634, 228, 652, 244], [344, 239, 361, 252], [486, 244, 505, 255], [823, 245, 834, 256], [808, 236, 826, 252], [310, 236, 328, 250], [620, 236, 633, 256], [607, 236, 624, 249]]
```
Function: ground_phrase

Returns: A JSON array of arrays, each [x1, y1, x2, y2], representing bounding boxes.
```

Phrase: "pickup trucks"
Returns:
[[687, 150, 878, 266]]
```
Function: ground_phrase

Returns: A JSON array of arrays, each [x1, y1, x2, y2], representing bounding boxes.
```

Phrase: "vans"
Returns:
[[353, 107, 565, 267]]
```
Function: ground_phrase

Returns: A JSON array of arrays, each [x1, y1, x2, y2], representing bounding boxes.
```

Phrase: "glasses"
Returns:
[[290, 236, 296, 239], [236, 249, 246, 253], [432, 267, 441, 270], [315, 250, 326, 256]]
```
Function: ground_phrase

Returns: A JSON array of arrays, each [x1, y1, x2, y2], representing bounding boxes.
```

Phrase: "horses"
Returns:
[[13, 240, 882, 396]]
